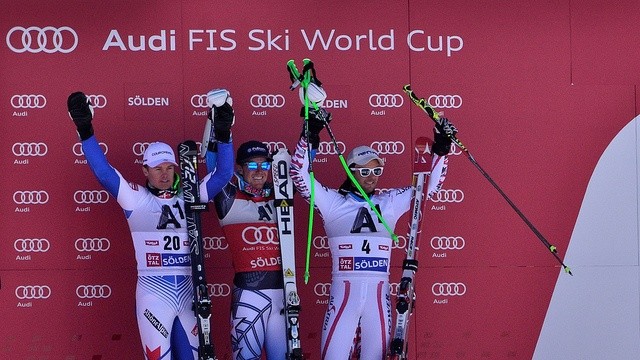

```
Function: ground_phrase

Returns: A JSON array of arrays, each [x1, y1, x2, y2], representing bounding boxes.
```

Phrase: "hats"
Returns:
[[143, 142, 179, 168], [236, 141, 274, 164], [347, 146, 384, 167]]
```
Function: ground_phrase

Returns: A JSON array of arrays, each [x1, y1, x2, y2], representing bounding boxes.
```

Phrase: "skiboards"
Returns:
[[269, 148, 302, 359], [177, 139, 217, 360], [383, 136, 435, 359]]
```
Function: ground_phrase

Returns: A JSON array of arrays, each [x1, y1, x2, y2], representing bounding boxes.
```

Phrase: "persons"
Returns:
[[69, 91, 234, 360], [200, 102, 332, 360], [289, 108, 458, 360]]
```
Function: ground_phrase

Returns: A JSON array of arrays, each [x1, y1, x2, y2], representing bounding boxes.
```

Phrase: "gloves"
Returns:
[[433, 117, 458, 156], [67, 92, 94, 140], [206, 89, 235, 143], [300, 107, 332, 148]]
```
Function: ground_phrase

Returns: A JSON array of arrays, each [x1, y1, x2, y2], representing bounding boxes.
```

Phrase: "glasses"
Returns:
[[241, 161, 271, 171], [350, 167, 384, 177]]
